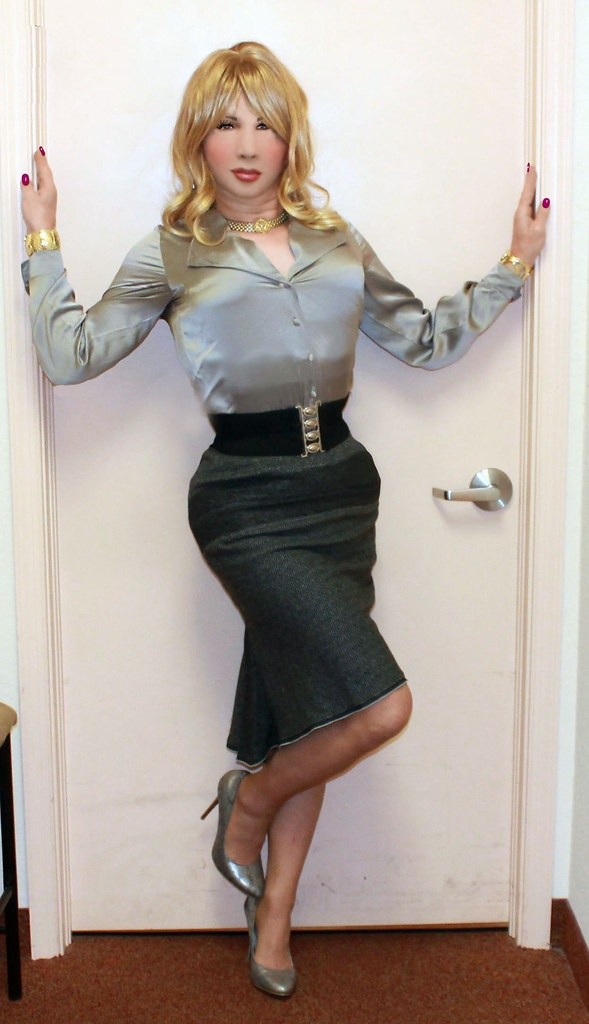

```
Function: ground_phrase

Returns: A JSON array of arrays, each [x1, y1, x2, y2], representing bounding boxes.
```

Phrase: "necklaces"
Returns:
[[213, 204, 301, 233]]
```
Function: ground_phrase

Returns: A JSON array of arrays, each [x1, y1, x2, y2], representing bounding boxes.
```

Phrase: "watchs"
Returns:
[[497, 252, 532, 286]]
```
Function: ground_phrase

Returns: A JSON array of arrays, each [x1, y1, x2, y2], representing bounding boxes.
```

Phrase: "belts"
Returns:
[[208, 391, 351, 457]]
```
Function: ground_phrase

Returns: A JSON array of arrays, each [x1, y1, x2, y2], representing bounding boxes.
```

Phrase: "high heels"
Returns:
[[201, 770, 266, 899], [244, 895, 296, 997]]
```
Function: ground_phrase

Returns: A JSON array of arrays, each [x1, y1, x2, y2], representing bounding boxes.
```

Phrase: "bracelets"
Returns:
[[23, 226, 60, 260]]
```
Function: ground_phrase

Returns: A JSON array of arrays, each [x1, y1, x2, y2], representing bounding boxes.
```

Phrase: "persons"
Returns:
[[20, 40, 551, 1004]]
